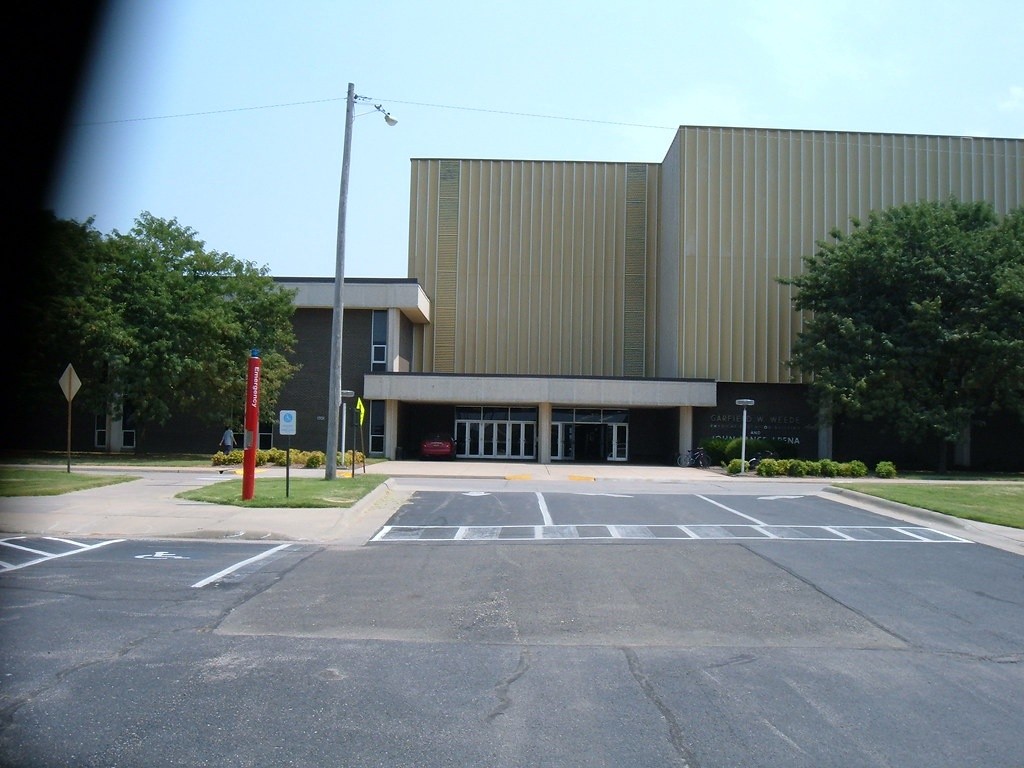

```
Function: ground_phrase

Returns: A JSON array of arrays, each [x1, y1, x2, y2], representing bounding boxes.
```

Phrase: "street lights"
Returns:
[[328, 99, 396, 478], [736, 399, 755, 472], [340, 390, 356, 464]]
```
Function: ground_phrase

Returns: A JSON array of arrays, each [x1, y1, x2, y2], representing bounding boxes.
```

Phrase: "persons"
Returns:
[[219, 424, 237, 455]]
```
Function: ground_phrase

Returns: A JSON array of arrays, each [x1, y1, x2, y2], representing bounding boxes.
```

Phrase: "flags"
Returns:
[[356, 397, 365, 426]]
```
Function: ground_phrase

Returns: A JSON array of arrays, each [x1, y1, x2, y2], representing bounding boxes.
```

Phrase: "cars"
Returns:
[[420, 432, 459, 460]]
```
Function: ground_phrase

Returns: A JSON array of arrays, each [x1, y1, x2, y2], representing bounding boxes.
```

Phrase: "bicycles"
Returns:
[[748, 450, 774, 469], [677, 447, 712, 469]]
